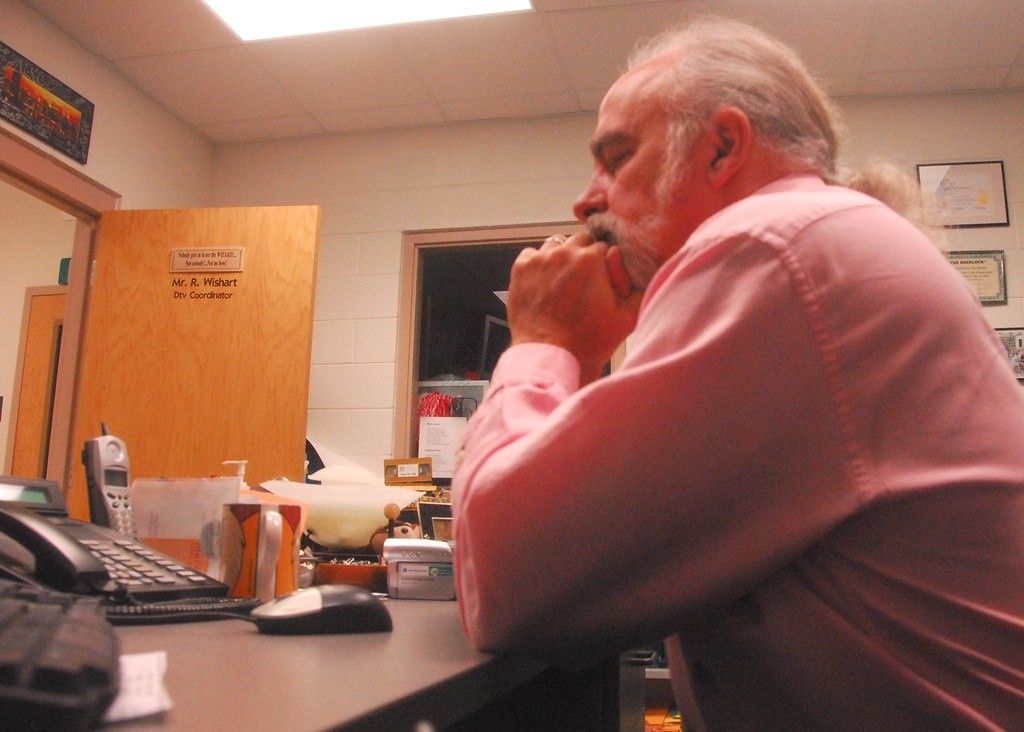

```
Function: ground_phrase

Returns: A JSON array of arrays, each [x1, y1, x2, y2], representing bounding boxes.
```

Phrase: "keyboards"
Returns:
[[0, 599, 120, 732]]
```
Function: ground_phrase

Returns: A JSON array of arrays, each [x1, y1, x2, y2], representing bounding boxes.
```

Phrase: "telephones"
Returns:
[[0, 476, 231, 618], [81, 422, 137, 541]]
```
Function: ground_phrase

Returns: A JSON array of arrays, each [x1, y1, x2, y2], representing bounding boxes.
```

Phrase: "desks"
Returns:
[[99, 594, 658, 732]]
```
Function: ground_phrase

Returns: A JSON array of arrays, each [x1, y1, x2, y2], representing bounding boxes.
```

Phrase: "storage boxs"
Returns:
[[144, 484, 306, 599], [385, 457, 432, 487]]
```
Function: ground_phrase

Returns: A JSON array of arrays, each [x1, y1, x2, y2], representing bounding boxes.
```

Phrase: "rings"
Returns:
[[543, 236, 568, 246]]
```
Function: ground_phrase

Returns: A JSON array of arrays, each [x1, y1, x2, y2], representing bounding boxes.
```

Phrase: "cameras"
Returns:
[[383, 537, 456, 601]]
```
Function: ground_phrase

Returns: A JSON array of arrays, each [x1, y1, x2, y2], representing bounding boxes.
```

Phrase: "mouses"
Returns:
[[250, 582, 392, 636]]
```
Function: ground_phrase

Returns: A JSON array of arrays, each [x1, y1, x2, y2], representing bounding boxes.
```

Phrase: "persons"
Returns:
[[451, 21, 1024, 732]]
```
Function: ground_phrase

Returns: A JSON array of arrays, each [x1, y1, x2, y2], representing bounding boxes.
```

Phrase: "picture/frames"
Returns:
[[0, 41, 95, 164]]
